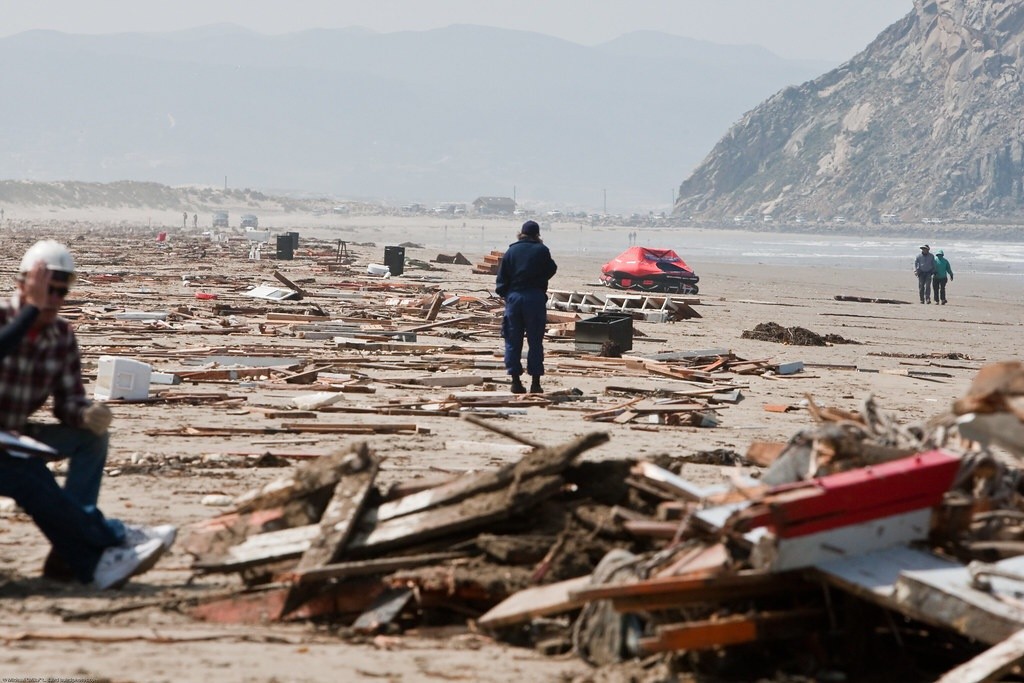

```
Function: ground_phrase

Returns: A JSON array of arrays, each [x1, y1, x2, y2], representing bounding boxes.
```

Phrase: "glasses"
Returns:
[[48, 285, 69, 297]]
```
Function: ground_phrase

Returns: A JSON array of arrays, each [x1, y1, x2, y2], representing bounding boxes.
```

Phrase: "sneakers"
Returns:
[[511, 381, 526, 394], [92, 539, 165, 592], [530, 381, 544, 393], [125, 524, 179, 555]]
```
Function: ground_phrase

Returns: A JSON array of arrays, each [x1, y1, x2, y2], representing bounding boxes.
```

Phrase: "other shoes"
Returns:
[[927, 301, 931, 304], [941, 299, 947, 305], [920, 301, 924, 304], [935, 301, 939, 305]]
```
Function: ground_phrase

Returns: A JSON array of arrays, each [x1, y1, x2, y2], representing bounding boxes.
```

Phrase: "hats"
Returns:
[[920, 244, 930, 250], [522, 221, 539, 235], [936, 249, 944, 255]]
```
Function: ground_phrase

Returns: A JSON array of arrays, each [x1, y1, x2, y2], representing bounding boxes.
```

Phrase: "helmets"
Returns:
[[17, 240, 76, 274]]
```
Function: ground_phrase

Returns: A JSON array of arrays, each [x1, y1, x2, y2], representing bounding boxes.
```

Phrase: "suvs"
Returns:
[[547, 209, 561, 217], [212, 212, 228, 227]]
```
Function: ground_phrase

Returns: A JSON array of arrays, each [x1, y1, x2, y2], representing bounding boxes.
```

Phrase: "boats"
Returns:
[[598, 246, 700, 295]]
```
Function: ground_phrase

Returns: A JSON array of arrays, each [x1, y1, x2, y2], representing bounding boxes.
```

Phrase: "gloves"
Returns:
[[950, 276, 953, 281]]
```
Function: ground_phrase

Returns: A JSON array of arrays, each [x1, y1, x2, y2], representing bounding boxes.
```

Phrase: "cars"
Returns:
[[922, 217, 943, 225], [514, 208, 525, 216], [239, 213, 258, 229], [334, 206, 349, 212], [817, 217, 826, 223], [833, 217, 846, 224], [763, 215, 773, 223], [734, 216, 744, 223]]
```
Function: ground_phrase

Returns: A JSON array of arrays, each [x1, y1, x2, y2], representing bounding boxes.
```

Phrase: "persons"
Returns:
[[494, 219, 559, 395], [0, 237, 182, 592], [913, 244, 935, 304], [932, 248, 954, 305]]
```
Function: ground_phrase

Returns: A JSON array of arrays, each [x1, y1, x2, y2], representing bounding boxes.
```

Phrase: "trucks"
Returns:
[[881, 214, 903, 225]]
